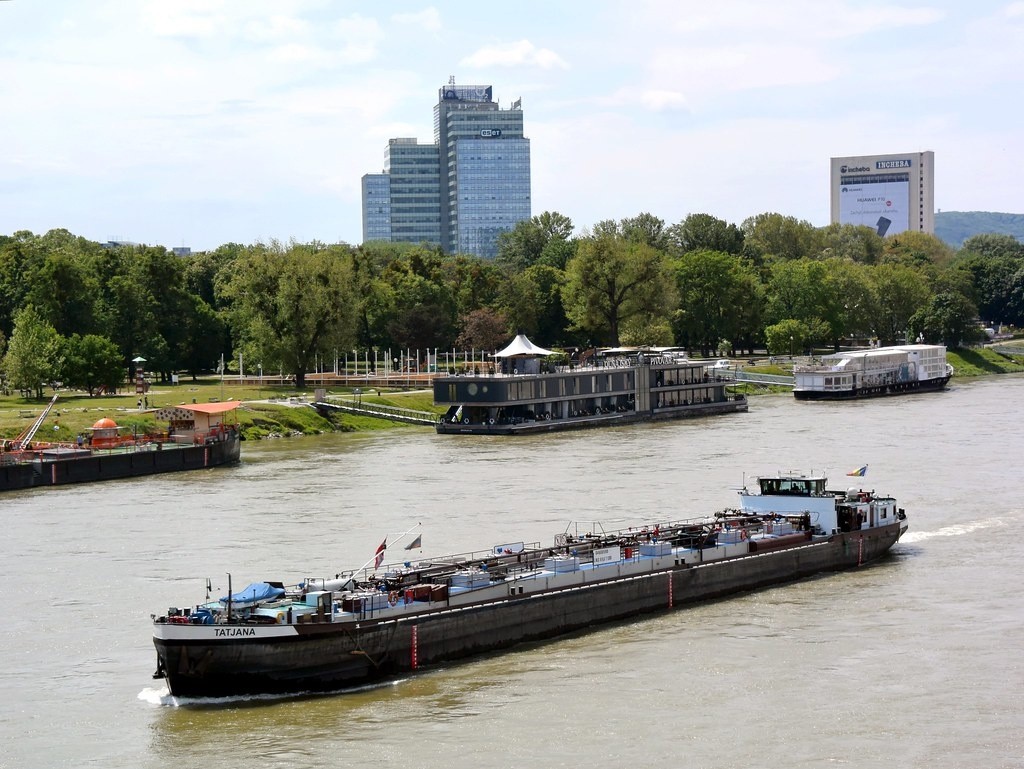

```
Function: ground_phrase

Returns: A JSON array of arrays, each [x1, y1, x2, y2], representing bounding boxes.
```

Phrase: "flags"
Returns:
[[404, 534, 422, 550], [846, 464, 868, 476], [375, 537, 387, 570]]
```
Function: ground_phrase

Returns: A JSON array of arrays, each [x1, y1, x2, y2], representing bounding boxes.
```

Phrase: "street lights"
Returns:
[[790, 335, 793, 355], [487, 353, 491, 363], [392, 357, 399, 372], [257, 359, 263, 385]]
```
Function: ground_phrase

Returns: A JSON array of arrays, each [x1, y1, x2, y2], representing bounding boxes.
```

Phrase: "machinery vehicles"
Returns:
[[401, 358, 420, 373]]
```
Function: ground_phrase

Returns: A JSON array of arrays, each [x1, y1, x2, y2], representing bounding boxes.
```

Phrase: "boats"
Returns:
[[433, 351, 749, 436], [789, 342, 954, 400], [150, 470, 908, 702], [0, 399, 247, 493]]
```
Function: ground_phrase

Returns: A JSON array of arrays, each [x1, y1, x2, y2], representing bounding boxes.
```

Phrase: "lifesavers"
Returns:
[[275, 611, 285, 624], [389, 590, 399, 607], [489, 418, 494, 424], [545, 414, 549, 420], [441, 418, 445, 424], [688, 400, 691, 405], [514, 368, 518, 374], [682, 379, 685, 384], [741, 529, 748, 540], [464, 417, 469, 424], [573, 411, 577, 416]]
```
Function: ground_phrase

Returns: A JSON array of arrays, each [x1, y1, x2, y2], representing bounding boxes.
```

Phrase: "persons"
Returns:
[[52, 382, 59, 391], [137, 395, 148, 410], [654, 526, 659, 538], [790, 483, 800, 491], [769, 356, 776, 365], [748, 358, 755, 366], [77, 433, 92, 448], [168, 424, 176, 442], [145, 380, 150, 392], [704, 372, 709, 383], [213, 611, 222, 624], [482, 560, 487, 571]]
